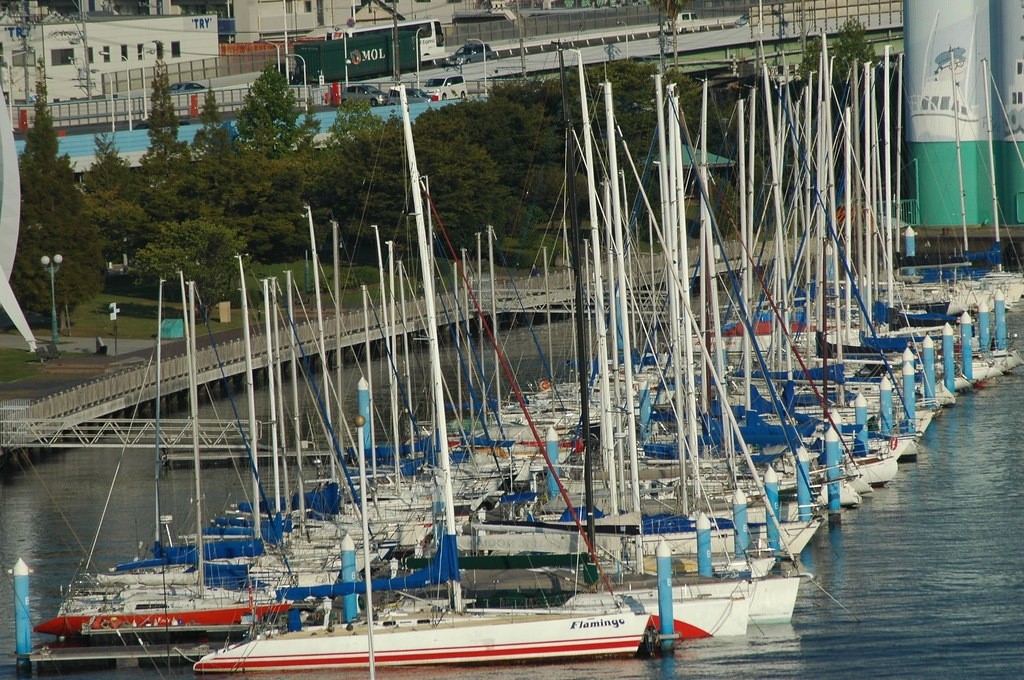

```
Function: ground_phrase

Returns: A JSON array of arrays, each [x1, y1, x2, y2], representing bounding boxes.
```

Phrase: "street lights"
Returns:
[[334, 22, 351, 86], [0, 49, 34, 131], [68, 56, 87, 78], [286, 54, 308, 115], [141, 39, 160, 120], [615, 20, 628, 60], [97, 51, 133, 131], [37, 254, 63, 344], [263, 37, 297, 74], [92, 68, 115, 133], [467, 39, 488, 102], [415, 27, 423, 93]]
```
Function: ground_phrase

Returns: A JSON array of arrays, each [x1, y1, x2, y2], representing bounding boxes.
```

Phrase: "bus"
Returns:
[[325, 18, 447, 66]]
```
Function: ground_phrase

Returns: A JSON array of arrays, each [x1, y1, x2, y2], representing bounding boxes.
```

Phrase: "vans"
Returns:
[[421, 74, 468, 101]]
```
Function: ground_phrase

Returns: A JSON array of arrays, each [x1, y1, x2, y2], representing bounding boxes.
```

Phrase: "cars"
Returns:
[[161, 81, 205, 95], [260, 63, 294, 83], [735, 13, 750, 28], [134, 121, 191, 130], [387, 86, 432, 106]]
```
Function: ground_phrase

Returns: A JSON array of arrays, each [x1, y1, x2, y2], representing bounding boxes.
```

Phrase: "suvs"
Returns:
[[665, 12, 704, 34], [444, 42, 494, 66], [341, 84, 389, 107]]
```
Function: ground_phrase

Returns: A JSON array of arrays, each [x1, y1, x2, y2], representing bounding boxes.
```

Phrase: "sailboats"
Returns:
[[26, 0, 1024, 677]]
